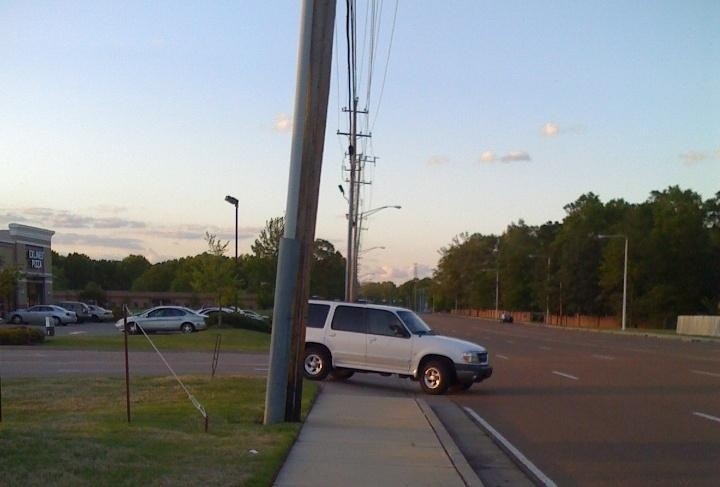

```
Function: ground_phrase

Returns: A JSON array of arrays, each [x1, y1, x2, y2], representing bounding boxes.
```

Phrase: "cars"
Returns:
[[9, 298, 267, 335], [501, 312, 513, 322]]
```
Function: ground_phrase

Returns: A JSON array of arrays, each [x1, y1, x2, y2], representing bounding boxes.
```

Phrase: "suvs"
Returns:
[[301, 296, 492, 394]]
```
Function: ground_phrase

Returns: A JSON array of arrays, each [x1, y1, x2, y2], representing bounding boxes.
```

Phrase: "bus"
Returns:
[[358, 298, 368, 304]]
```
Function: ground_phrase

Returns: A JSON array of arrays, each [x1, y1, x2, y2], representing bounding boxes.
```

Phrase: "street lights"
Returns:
[[349, 203, 402, 297], [528, 252, 551, 325], [493, 239, 501, 322], [596, 231, 629, 330], [222, 194, 239, 312]]
